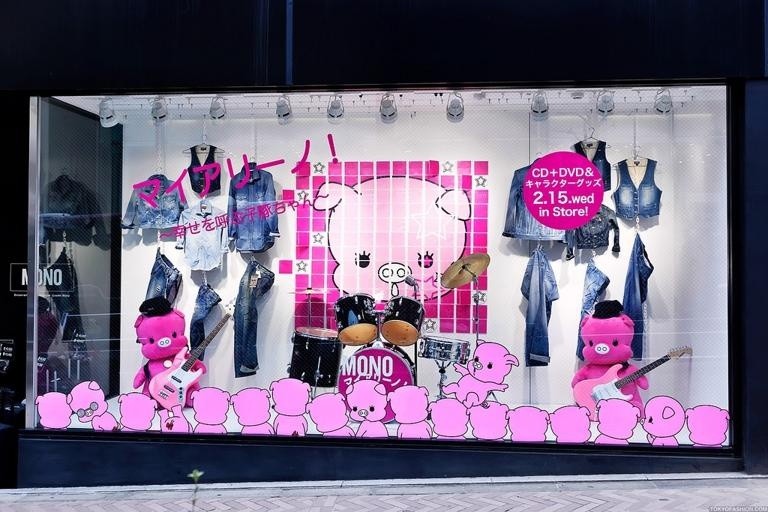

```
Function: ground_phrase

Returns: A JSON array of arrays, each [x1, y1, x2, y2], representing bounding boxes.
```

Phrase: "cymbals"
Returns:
[[440, 252, 490, 288], [288, 290, 331, 295]]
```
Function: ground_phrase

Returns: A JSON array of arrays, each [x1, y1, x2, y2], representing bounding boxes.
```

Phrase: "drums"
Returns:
[[339, 341, 417, 424], [289, 327, 342, 388], [381, 296, 425, 346], [422, 336, 470, 365], [333, 293, 379, 346]]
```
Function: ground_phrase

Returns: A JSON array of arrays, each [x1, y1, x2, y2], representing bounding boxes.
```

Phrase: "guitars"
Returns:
[[572, 345, 692, 422], [148, 297, 237, 412]]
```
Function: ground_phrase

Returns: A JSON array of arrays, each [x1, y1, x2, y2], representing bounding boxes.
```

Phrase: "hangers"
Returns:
[[574, 126, 650, 170], [184, 135, 227, 155]]
